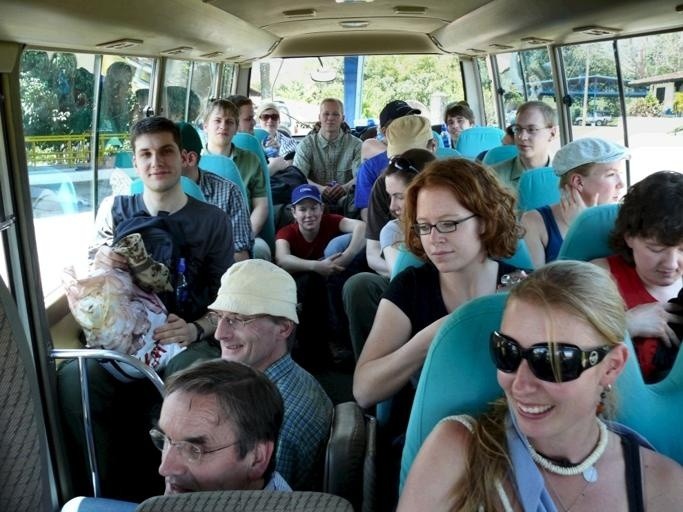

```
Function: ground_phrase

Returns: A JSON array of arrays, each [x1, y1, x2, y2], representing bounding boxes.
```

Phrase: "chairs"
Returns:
[[277, 120, 378, 142], [323, 401, 375, 512], [128, 128, 274, 260], [135, 489, 354, 512], [375, 125, 682, 496]]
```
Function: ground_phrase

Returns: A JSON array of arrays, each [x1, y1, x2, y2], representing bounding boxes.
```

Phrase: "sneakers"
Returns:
[[330, 343, 353, 364]]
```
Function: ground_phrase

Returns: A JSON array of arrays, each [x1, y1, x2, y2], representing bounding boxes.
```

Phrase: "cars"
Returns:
[[575, 110, 613, 126]]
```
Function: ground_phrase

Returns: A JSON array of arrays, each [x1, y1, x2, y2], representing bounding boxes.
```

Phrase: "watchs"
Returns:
[[189, 320, 204, 344]]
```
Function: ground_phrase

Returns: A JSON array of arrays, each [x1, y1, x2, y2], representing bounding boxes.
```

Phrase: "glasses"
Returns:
[[205, 311, 261, 330], [447, 100, 469, 110], [260, 114, 280, 122], [489, 329, 615, 383], [388, 155, 419, 173], [410, 214, 478, 236], [148, 427, 244, 466], [512, 124, 547, 136]]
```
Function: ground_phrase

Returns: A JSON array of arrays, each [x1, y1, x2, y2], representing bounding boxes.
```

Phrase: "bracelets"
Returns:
[[203, 309, 218, 334]]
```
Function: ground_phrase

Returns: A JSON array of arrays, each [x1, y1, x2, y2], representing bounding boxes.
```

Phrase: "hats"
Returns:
[[258, 101, 278, 114], [553, 137, 631, 176], [380, 100, 422, 128], [208, 258, 299, 324], [385, 115, 433, 159], [292, 183, 322, 207]]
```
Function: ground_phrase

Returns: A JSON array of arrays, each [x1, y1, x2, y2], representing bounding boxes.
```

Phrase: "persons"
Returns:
[[171, 120, 254, 264], [157, 356, 295, 494], [56, 117, 233, 486], [213, 256, 333, 494], [519, 137, 632, 269], [205, 94, 476, 367], [475, 123, 515, 165], [351, 158, 535, 512], [394, 258, 683, 511], [584, 170, 683, 385], [489, 100, 558, 190], [18, 48, 202, 139]]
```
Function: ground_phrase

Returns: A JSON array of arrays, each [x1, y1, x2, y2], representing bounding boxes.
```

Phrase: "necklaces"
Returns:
[[520, 416, 608, 477], [528, 464, 606, 512]]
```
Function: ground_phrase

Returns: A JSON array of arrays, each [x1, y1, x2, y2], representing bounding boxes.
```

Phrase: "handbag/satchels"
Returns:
[[264, 165, 308, 206]]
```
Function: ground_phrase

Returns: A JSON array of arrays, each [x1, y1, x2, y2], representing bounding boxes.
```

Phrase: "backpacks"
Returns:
[[109, 210, 184, 314]]
